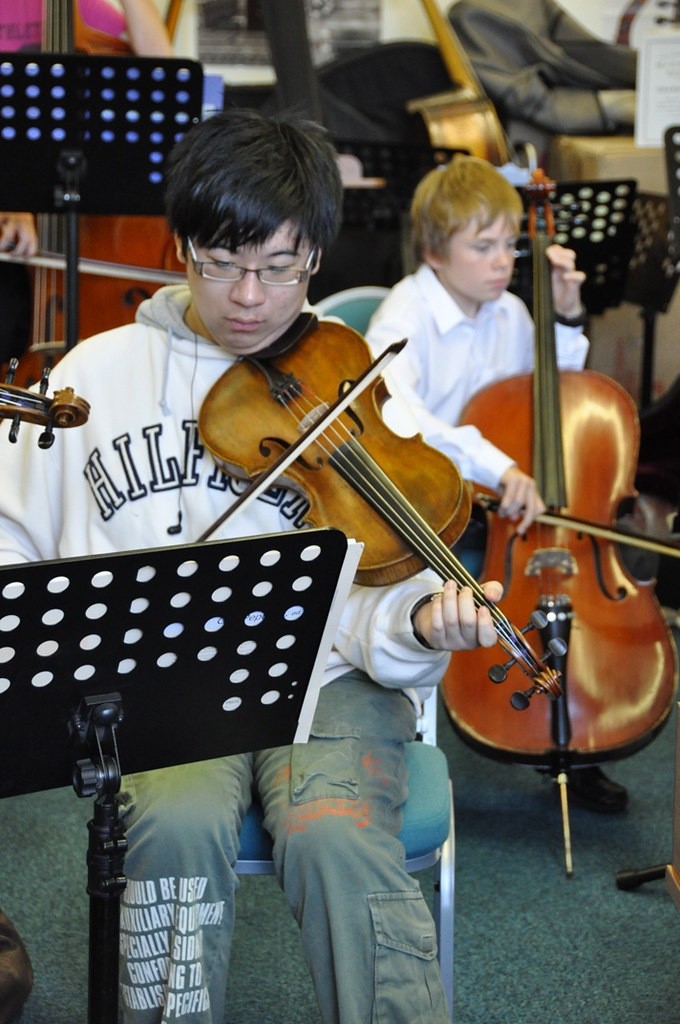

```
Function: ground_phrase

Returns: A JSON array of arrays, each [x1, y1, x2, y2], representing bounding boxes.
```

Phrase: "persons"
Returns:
[[0, 109, 505, 1024], [0, 0, 638, 161], [360, 153, 630, 817]]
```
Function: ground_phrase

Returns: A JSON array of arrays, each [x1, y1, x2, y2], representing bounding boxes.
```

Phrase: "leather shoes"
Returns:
[[553, 765, 629, 812]]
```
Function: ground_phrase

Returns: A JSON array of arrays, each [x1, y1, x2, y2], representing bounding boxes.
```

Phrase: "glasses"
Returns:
[[185, 233, 318, 286]]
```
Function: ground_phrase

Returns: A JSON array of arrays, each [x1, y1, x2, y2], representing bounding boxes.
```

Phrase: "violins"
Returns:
[[0, 352, 91, 451], [197, 311, 569, 712]]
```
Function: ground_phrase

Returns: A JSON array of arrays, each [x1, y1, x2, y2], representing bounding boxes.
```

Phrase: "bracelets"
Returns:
[[554, 305, 588, 328]]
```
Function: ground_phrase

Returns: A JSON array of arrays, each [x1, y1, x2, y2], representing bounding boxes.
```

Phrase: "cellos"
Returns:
[[409, 0, 511, 170], [438, 140, 673, 879], [6, 0, 193, 405]]
